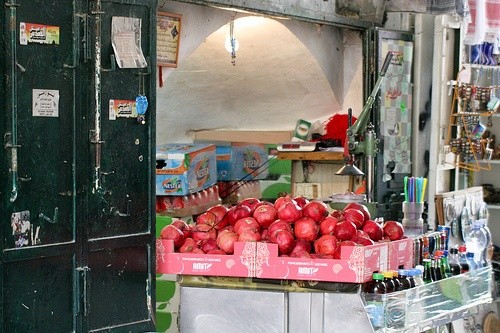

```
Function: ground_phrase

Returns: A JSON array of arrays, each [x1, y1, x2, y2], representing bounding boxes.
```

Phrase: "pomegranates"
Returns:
[[159, 193, 405, 261]]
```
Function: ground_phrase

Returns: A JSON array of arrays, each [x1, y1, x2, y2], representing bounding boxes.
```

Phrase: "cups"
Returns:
[[401, 201, 424, 228]]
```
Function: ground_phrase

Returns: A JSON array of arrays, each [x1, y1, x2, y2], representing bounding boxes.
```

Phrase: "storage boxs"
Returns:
[[155, 131, 414, 333]]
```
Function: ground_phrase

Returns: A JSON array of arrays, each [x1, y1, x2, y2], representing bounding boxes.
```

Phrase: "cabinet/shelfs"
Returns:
[[455, 27, 500, 266]]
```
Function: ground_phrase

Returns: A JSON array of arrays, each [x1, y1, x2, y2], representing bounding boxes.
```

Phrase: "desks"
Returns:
[[278, 152, 356, 191]]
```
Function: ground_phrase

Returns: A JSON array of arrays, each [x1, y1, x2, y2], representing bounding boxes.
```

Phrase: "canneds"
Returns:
[[154, 179, 260, 212]]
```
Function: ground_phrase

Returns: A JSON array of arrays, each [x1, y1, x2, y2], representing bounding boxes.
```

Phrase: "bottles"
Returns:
[[364, 218, 491, 329]]
